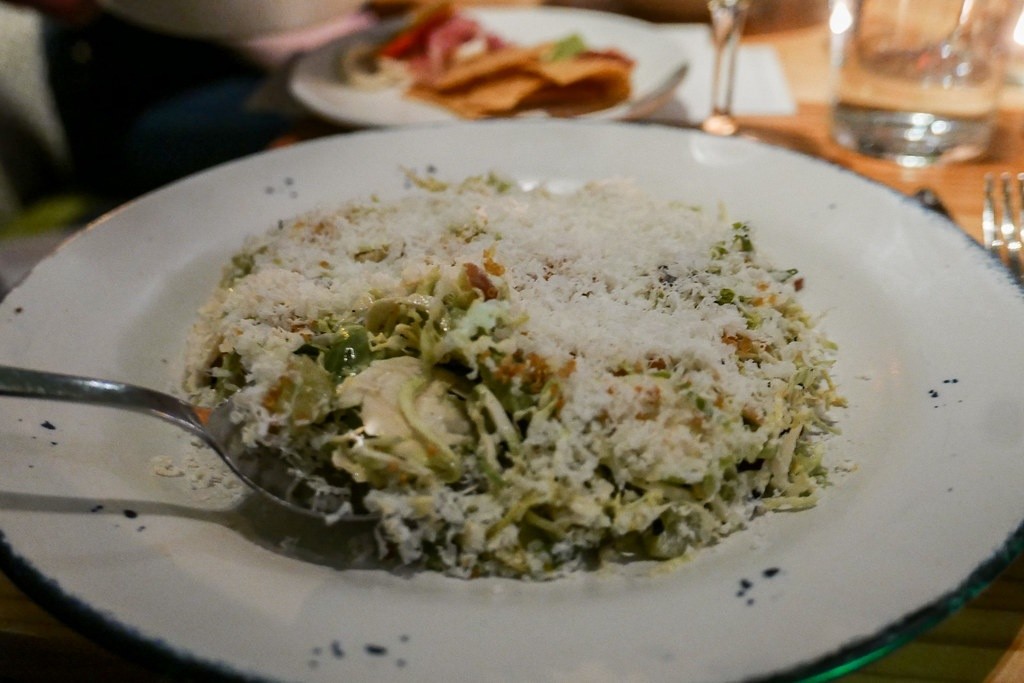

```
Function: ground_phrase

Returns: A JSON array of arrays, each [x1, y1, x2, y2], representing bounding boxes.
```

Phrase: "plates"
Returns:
[[285, 7, 691, 128], [0, 119, 1024, 683]]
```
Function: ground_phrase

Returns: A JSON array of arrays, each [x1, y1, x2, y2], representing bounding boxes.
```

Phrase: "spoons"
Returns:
[[0, 363, 380, 522]]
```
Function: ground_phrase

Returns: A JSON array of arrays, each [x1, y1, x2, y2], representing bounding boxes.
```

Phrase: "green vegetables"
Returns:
[[193, 223, 842, 578]]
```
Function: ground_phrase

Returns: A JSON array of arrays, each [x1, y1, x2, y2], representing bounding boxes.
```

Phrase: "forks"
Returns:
[[979, 170, 1024, 293]]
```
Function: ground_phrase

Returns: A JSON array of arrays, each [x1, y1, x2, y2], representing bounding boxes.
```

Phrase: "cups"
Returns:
[[826, 0, 1024, 168]]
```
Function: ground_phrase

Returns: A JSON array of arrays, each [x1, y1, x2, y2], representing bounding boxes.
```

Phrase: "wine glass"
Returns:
[[703, 0, 755, 137]]
